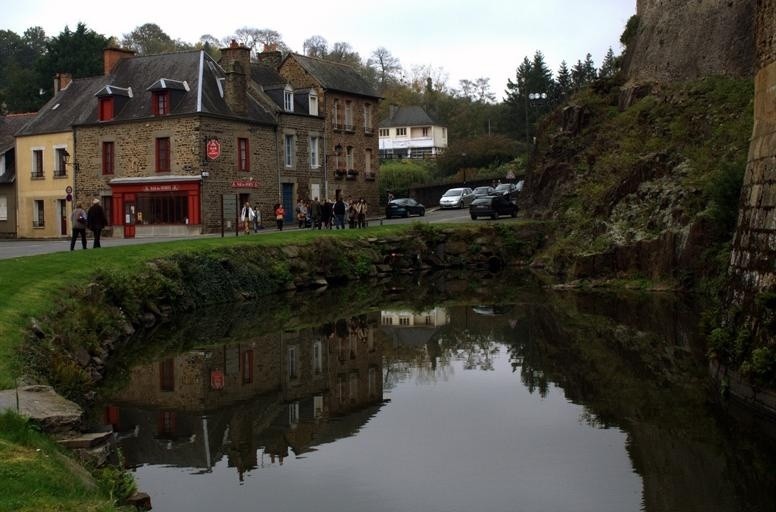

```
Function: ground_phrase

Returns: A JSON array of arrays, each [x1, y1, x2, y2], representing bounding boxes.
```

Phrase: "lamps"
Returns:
[[61, 150, 79, 173]]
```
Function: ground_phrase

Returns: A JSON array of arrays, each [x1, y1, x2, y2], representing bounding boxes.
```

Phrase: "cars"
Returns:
[[489, 190, 511, 196], [495, 183, 517, 192], [469, 196, 520, 220], [516, 180, 524, 191], [473, 186, 495, 199], [385, 198, 426, 219]]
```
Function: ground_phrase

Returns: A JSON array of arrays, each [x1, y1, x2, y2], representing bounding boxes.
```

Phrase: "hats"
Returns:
[[92, 199, 100, 204]]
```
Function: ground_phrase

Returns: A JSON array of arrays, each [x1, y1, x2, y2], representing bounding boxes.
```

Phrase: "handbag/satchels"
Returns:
[[79, 216, 84, 222]]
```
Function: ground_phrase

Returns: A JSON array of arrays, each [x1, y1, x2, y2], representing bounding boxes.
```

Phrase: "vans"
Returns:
[[439, 187, 477, 210]]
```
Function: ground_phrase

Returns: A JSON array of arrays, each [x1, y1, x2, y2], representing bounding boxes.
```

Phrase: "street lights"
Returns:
[[528, 92, 547, 121]]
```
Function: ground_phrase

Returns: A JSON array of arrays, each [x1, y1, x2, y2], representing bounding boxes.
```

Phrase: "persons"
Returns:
[[241, 201, 256, 234], [87, 198, 108, 247], [253, 206, 261, 233], [296, 196, 368, 231], [70, 200, 88, 251], [276, 205, 285, 231], [320, 314, 369, 344], [384, 191, 394, 211]]
